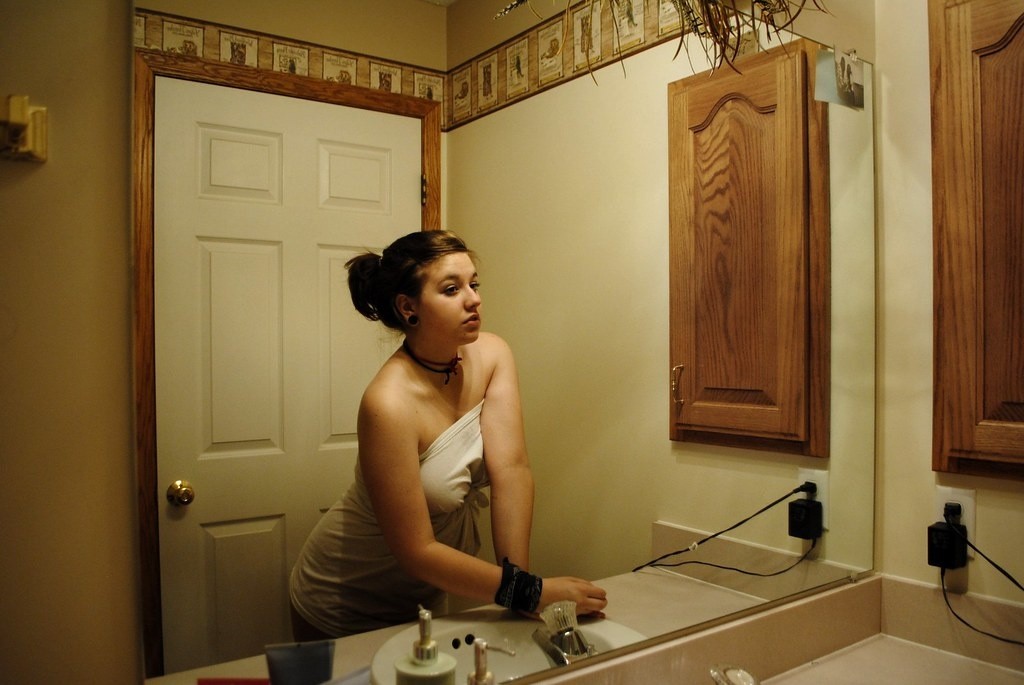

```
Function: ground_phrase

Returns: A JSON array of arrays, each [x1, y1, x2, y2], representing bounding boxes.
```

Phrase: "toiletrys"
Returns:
[[469, 639, 515, 685], [264, 639, 337, 685], [395, 604, 456, 685]]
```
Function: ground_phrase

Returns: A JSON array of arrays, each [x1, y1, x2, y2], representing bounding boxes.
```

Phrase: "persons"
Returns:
[[290, 229, 608, 644]]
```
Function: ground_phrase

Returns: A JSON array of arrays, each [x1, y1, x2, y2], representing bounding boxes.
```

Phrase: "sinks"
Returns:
[[371, 605, 647, 685]]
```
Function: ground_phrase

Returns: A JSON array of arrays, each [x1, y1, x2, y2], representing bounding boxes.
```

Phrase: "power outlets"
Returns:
[[933, 482, 976, 561], [797, 465, 831, 532]]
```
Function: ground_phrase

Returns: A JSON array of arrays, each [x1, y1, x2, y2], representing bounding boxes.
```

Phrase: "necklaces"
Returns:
[[402, 339, 462, 385]]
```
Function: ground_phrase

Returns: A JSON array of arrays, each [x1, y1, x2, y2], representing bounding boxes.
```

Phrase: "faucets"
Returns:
[[531, 600, 595, 665]]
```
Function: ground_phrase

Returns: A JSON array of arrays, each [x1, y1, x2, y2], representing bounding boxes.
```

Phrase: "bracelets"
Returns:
[[494, 557, 543, 615]]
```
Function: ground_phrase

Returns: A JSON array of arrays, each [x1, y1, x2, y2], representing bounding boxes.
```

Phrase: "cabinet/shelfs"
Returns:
[[927, 0, 1024, 483], [665, 36, 831, 460]]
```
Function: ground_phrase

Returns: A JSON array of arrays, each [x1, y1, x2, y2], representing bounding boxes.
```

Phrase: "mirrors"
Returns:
[[130, 0, 876, 685]]
